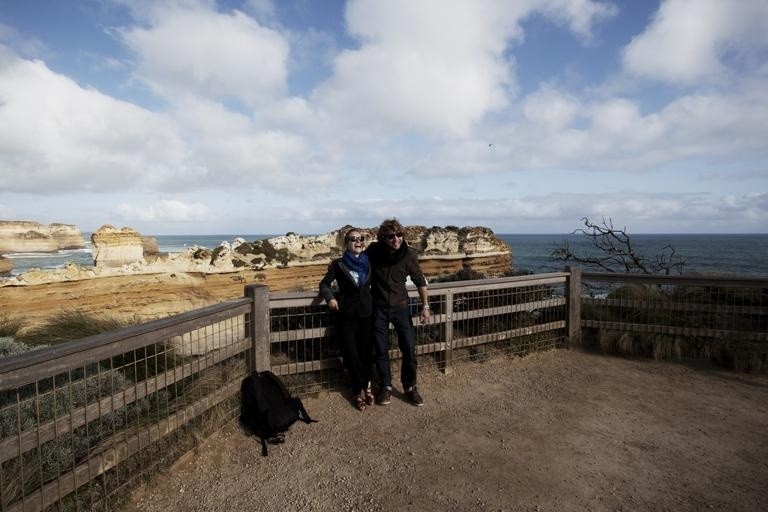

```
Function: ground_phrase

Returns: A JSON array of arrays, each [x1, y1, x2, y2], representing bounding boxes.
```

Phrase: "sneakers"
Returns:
[[404, 386, 425, 407], [377, 383, 393, 405]]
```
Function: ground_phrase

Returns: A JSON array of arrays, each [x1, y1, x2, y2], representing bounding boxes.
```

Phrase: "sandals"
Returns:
[[353, 387, 375, 412]]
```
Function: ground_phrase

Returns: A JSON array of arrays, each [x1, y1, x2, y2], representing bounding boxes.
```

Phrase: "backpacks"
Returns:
[[239, 368, 319, 457]]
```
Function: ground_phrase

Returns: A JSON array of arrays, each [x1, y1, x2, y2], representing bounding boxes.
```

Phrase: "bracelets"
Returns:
[[422, 304, 430, 309]]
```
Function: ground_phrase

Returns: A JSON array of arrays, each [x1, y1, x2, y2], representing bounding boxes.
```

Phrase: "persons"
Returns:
[[327, 218, 431, 408], [318, 227, 375, 412]]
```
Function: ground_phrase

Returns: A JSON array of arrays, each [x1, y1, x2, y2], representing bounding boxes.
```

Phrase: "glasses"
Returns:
[[347, 235, 365, 242], [385, 232, 403, 239]]
